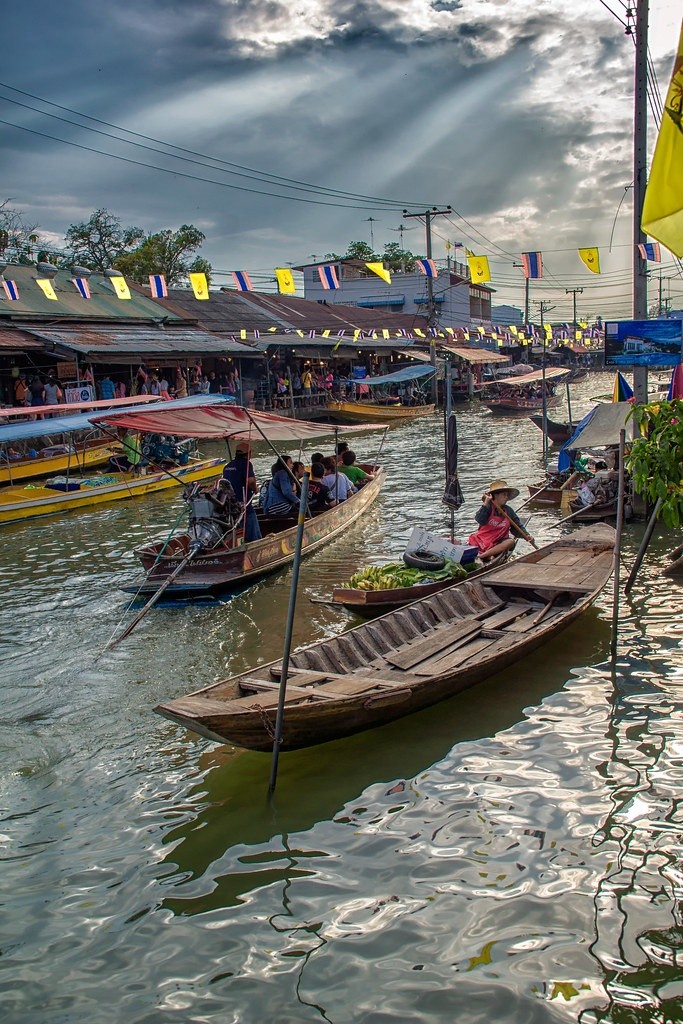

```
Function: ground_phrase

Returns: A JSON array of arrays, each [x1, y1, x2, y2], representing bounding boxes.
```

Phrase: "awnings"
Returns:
[[533, 346, 590, 355], [246, 336, 509, 364], [0, 325, 266, 358]]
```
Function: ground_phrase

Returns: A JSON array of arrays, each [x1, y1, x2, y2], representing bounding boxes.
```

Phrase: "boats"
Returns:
[[473, 363, 678, 522], [87, 403, 391, 603], [308, 515, 533, 621], [315, 364, 438, 423], [0, 395, 223, 527], [149, 520, 622, 756]]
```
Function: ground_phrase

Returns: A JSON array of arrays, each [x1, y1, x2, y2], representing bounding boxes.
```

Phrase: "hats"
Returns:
[[236, 443, 252, 454], [482, 481, 520, 502]]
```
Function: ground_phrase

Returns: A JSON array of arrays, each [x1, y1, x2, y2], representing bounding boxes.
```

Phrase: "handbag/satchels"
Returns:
[[56, 386, 62, 399]]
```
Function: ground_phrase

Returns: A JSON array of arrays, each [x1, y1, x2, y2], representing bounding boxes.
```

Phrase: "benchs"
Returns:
[[270, 665, 404, 689], [240, 678, 348, 700], [253, 483, 366, 538]]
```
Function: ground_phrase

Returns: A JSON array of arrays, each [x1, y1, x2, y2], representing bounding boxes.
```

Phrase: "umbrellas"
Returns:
[[612, 370, 633, 403], [442, 415, 465, 544], [667, 363, 683, 400]]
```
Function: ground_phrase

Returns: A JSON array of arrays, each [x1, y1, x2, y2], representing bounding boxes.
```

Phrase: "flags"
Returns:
[[237, 323, 602, 345], [578, 248, 600, 273], [638, 243, 661, 263], [417, 251, 543, 285], [3, 276, 131, 299], [642, 17, 683, 259], [149, 270, 254, 299], [275, 263, 391, 294]]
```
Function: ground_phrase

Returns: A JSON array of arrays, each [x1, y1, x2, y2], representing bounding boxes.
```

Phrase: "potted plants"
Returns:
[[242, 383, 256, 400]]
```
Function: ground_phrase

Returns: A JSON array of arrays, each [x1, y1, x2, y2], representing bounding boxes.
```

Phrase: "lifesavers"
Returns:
[[403, 550, 445, 569]]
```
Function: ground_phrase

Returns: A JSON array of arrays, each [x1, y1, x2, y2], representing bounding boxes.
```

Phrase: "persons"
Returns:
[[13, 365, 498, 419], [223, 442, 377, 544], [508, 359, 558, 397], [107, 427, 183, 471], [573, 450, 615, 498], [468, 481, 535, 563]]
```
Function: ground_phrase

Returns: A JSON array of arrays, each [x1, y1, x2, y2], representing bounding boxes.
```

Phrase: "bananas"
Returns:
[[340, 566, 413, 590]]
